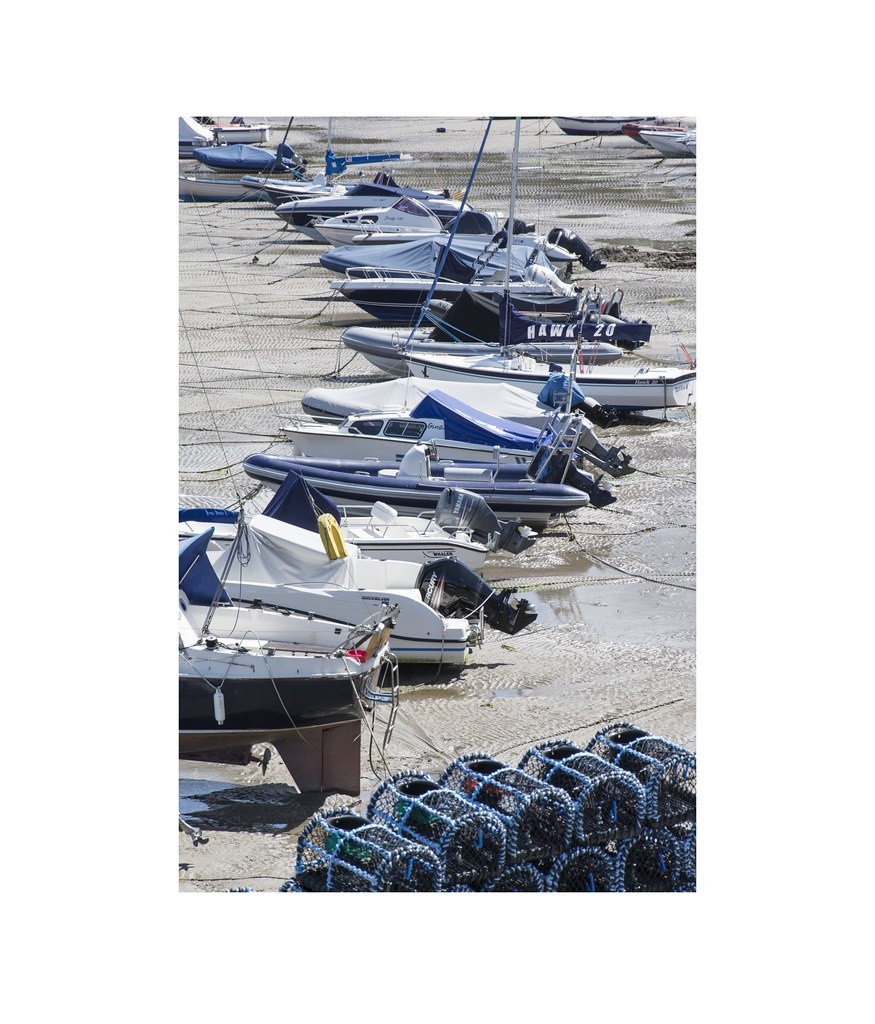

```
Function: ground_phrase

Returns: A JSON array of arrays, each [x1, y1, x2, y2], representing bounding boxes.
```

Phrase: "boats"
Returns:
[[179, 117, 696, 793]]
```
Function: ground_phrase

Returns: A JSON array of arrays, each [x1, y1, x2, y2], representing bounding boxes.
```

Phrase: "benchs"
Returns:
[[348, 500, 398, 539]]
